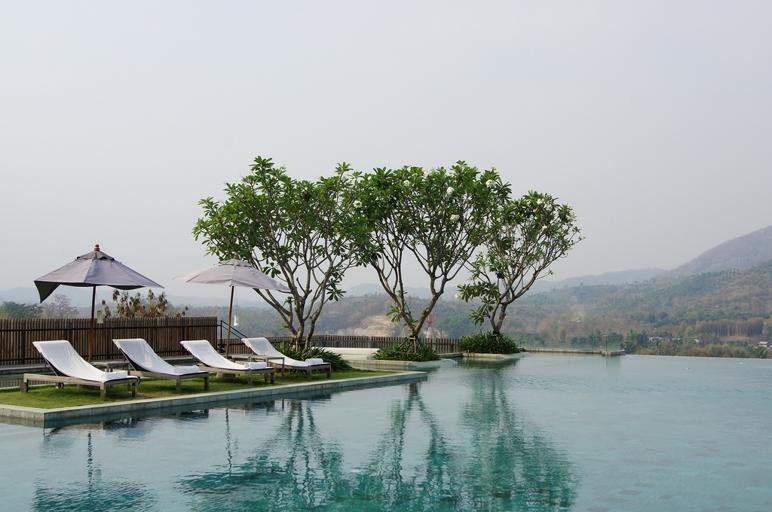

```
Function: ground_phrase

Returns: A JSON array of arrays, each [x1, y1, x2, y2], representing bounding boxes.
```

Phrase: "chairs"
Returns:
[[180, 339, 275, 384], [113, 338, 210, 396], [22, 340, 142, 401], [241, 337, 331, 380]]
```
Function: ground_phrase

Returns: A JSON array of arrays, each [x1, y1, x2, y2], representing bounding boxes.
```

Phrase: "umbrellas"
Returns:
[[34, 244, 163, 362], [186, 255, 292, 359]]
